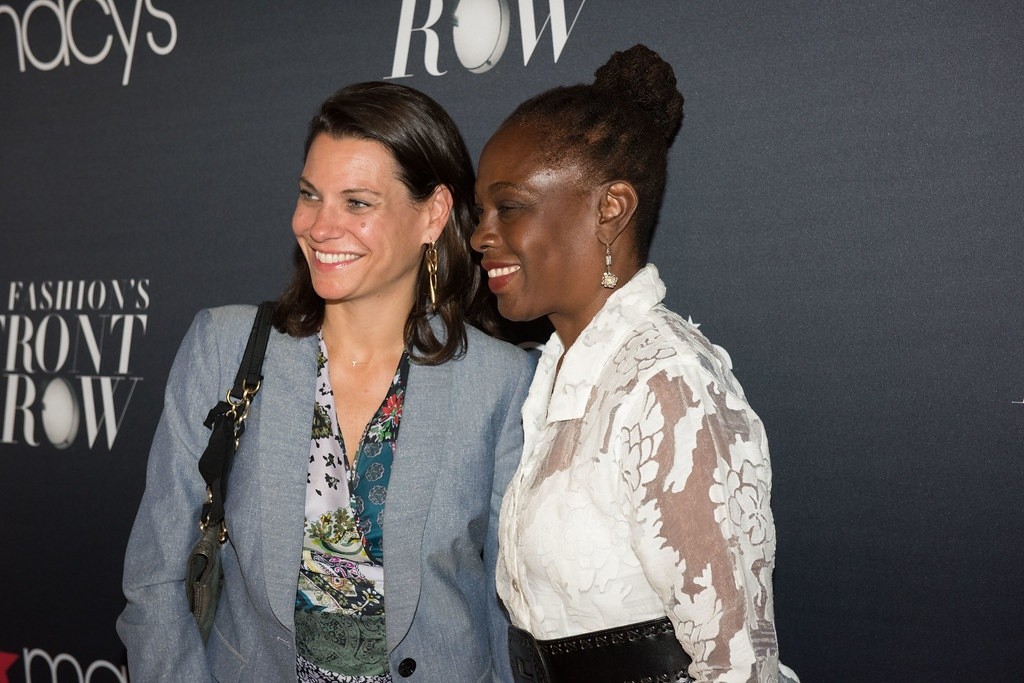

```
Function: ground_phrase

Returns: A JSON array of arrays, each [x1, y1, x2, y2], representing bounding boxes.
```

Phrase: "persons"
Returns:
[[116, 82, 536, 683], [471, 45, 799, 683]]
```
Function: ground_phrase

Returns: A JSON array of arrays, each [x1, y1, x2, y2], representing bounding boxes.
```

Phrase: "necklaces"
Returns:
[[324, 339, 403, 367]]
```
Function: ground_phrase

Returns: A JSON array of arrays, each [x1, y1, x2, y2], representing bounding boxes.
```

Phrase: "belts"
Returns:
[[507, 617, 692, 683]]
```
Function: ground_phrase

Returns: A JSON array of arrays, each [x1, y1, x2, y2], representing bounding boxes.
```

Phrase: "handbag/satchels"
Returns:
[[186, 302, 276, 647]]
